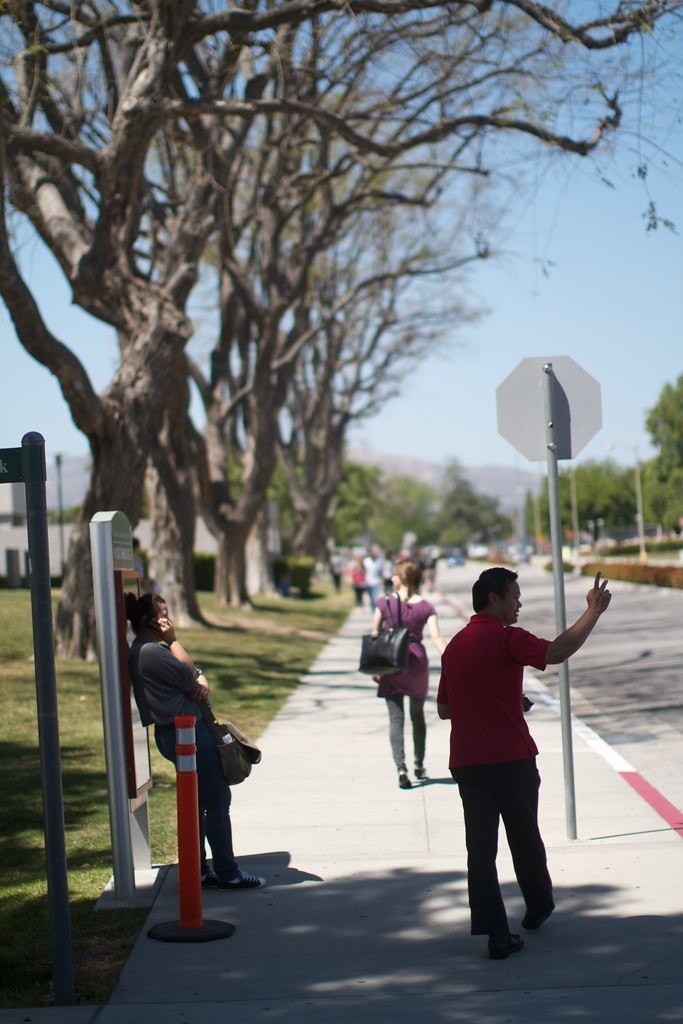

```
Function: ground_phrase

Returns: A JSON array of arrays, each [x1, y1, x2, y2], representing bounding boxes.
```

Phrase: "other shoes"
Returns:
[[415, 767, 425, 779], [399, 774, 411, 789]]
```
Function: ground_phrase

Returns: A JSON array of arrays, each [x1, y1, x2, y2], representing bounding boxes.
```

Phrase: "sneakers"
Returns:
[[200, 871, 218, 887], [217, 871, 265, 891]]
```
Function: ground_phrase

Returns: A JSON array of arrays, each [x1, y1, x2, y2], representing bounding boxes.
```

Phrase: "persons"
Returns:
[[128, 593, 265, 891], [437, 566, 612, 960], [330, 549, 435, 609], [371, 560, 445, 789]]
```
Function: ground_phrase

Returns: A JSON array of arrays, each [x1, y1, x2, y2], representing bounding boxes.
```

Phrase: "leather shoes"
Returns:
[[489, 933, 523, 960], [522, 911, 552, 929]]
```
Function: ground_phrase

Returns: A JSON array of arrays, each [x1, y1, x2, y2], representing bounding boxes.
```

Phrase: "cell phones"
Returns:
[[146, 618, 160, 631]]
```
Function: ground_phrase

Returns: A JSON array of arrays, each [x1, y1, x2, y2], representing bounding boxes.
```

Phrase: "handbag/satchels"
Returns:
[[211, 719, 261, 785], [358, 593, 409, 675]]
